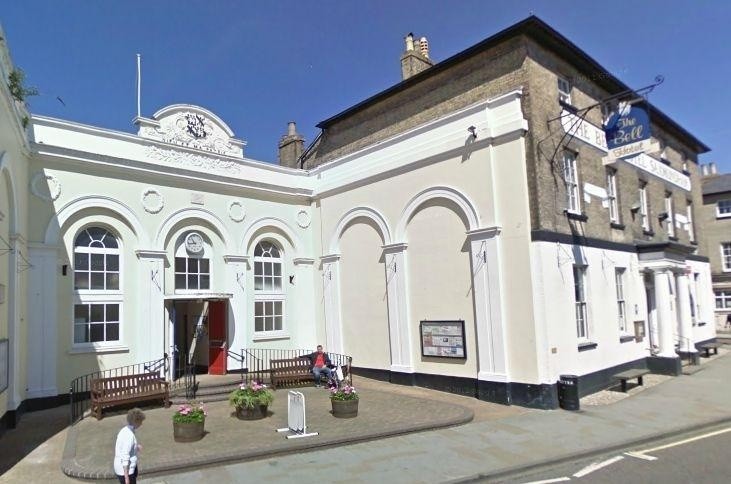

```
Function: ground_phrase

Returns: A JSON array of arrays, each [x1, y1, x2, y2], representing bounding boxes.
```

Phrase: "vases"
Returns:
[[235, 401, 266, 420], [331, 398, 359, 418], [173, 419, 204, 442]]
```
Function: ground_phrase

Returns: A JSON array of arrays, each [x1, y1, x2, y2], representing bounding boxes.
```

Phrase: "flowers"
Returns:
[[173, 402, 208, 425], [230, 380, 273, 408], [329, 380, 360, 400]]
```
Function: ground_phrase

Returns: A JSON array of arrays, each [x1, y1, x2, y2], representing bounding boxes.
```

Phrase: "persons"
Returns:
[[297, 345, 334, 388], [113, 407, 145, 484]]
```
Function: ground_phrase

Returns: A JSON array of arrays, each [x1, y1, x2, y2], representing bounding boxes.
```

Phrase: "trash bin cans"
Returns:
[[559, 374, 580, 411]]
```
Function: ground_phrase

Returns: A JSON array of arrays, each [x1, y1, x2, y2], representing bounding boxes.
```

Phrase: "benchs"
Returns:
[[269, 358, 337, 391], [612, 368, 650, 393], [90, 371, 170, 420], [700, 342, 722, 357]]
